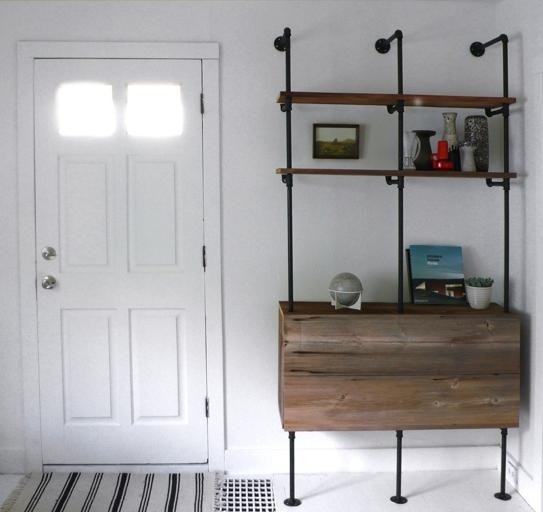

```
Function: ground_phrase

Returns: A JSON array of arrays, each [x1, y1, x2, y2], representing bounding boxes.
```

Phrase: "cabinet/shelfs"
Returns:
[[272, 25, 521, 509]]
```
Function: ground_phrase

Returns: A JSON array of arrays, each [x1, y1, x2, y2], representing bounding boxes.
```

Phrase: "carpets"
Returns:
[[0, 471, 276, 512]]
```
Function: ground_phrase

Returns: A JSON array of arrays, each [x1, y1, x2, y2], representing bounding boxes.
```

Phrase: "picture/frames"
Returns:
[[311, 123, 359, 159]]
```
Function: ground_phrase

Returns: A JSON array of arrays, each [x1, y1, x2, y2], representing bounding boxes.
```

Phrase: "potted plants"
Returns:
[[463, 276, 494, 311]]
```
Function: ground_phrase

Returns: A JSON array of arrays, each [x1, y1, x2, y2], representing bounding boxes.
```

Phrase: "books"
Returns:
[[409, 244, 468, 306]]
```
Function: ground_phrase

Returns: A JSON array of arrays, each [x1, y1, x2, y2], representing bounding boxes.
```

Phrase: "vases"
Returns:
[[441, 112, 460, 172], [457, 141, 477, 172], [410, 129, 437, 171], [463, 115, 489, 172]]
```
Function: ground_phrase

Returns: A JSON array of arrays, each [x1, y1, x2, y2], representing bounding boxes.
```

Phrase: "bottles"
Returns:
[[431, 139, 478, 173]]
